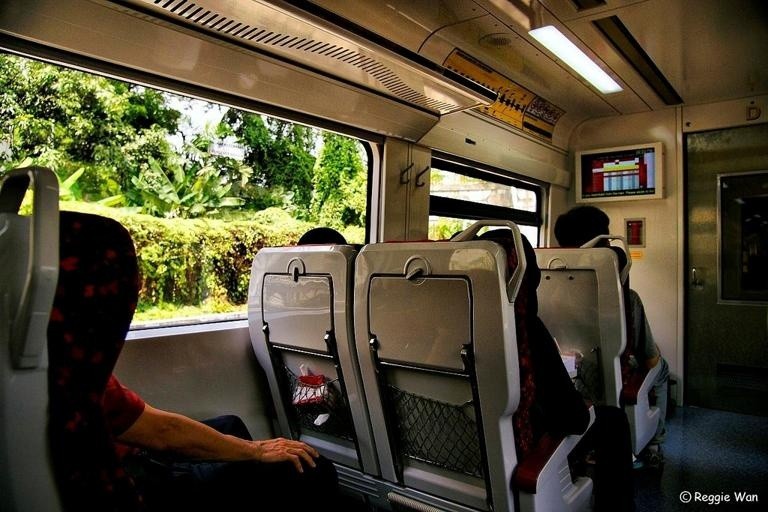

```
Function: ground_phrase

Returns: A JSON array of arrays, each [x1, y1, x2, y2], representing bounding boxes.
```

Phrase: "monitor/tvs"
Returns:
[[575, 141, 663, 202]]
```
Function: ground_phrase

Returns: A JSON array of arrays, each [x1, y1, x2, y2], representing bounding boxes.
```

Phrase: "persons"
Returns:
[[100, 370, 345, 512], [554, 201, 680, 421], [473, 228, 640, 512]]
